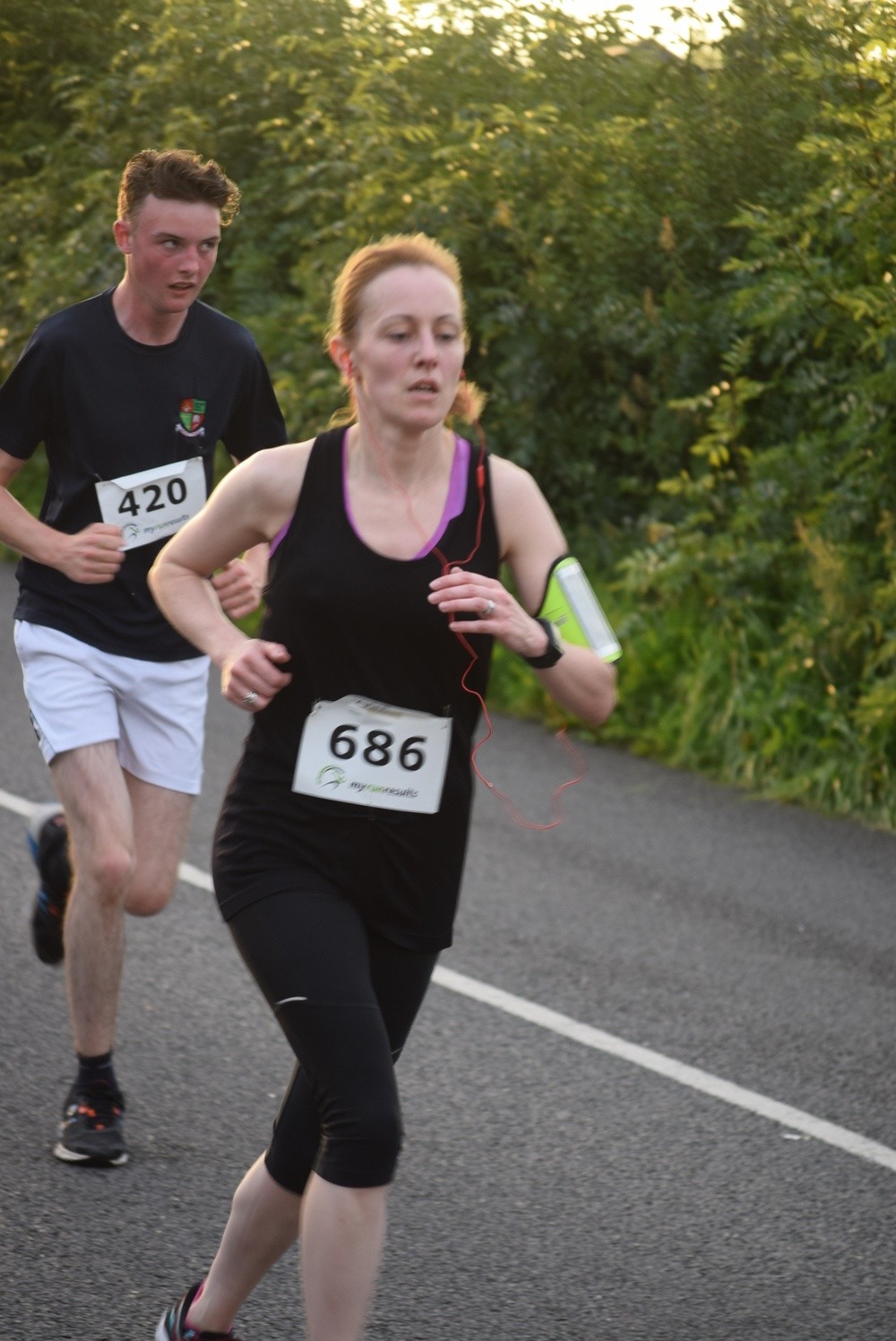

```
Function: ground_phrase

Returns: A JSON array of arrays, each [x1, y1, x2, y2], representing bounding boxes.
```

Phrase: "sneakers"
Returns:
[[54, 1080, 130, 1167], [156, 1278, 235, 1341], [26, 804, 72, 964]]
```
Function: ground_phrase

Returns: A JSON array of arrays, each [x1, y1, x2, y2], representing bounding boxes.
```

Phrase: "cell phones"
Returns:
[[554, 563, 623, 663]]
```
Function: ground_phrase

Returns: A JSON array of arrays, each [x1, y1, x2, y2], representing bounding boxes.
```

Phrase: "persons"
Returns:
[[0, 153, 287, 1158], [146, 232, 620, 1341]]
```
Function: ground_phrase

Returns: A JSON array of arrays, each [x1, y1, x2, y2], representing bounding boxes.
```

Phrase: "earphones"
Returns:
[[344, 359, 354, 379]]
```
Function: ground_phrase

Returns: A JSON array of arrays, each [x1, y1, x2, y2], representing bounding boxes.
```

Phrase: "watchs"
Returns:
[[520, 618, 566, 670]]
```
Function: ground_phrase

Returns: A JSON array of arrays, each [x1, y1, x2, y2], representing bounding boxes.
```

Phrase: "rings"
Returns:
[[481, 599, 494, 615], [241, 691, 260, 706]]
